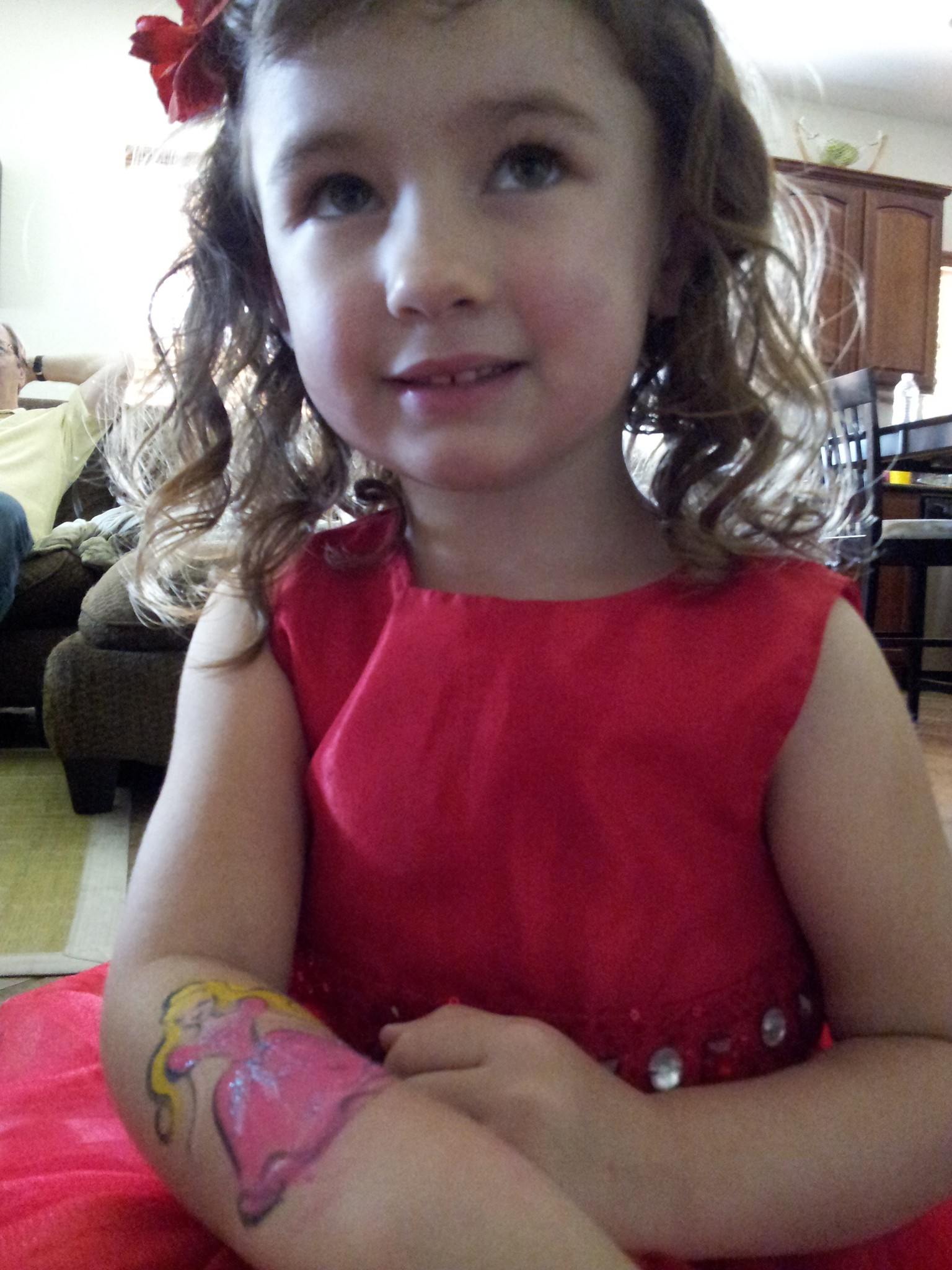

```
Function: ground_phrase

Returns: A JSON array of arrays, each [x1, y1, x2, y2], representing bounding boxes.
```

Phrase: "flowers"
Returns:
[[128, 0, 230, 123]]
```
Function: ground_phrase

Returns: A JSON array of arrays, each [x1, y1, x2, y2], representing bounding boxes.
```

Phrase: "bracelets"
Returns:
[[32, 355, 46, 382]]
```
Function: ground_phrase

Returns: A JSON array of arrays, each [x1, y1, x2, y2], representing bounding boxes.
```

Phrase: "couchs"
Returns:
[[0, 396, 236, 818]]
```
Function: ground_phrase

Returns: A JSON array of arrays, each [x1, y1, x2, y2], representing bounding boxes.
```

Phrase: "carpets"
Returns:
[[0, 741, 131, 976]]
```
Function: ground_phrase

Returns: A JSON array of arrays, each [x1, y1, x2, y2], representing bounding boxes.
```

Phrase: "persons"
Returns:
[[0, 0, 952, 1270], [0, 323, 135, 613]]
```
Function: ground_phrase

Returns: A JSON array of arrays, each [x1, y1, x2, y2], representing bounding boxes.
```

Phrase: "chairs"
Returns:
[[812, 361, 952, 721]]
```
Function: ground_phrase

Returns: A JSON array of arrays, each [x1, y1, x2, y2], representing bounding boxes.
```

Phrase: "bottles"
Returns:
[[891, 373, 920, 425]]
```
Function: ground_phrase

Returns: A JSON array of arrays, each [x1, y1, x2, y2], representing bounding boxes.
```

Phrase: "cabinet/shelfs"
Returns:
[[769, 153, 951, 394]]
